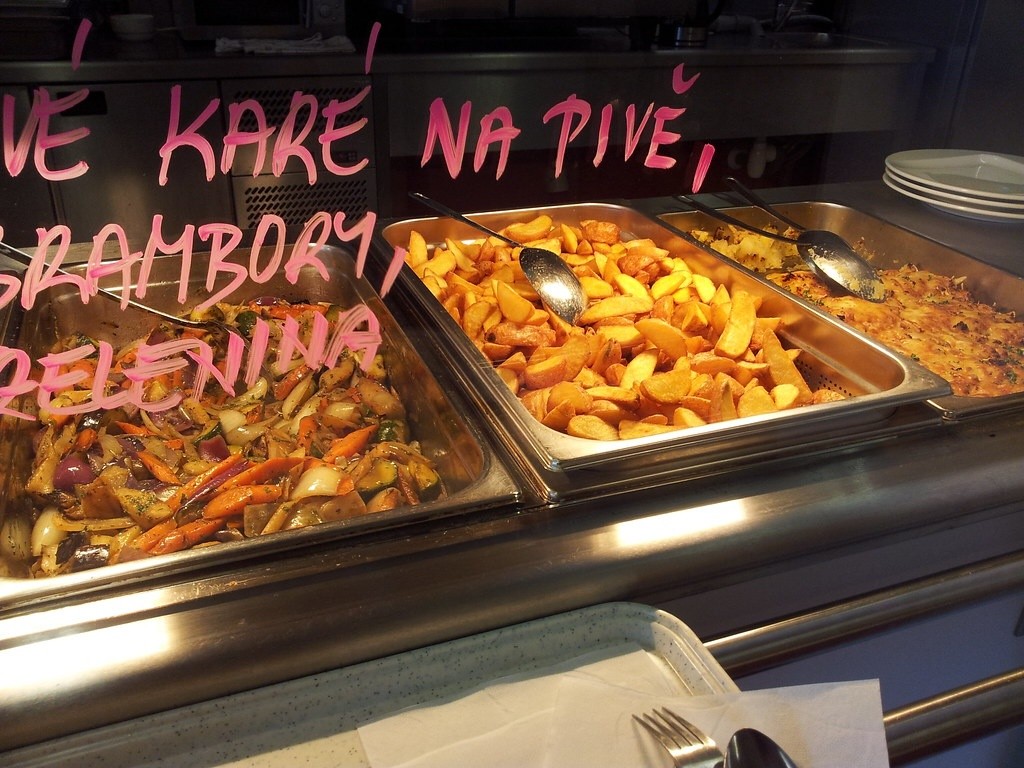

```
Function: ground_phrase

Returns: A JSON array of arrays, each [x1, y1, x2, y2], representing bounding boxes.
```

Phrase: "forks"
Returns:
[[632, 707, 725, 768]]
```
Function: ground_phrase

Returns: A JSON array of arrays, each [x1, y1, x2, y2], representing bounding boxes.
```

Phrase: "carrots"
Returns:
[[50, 303, 421, 559]]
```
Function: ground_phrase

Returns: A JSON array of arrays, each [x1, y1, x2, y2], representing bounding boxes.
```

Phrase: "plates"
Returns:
[[881, 148, 1024, 224]]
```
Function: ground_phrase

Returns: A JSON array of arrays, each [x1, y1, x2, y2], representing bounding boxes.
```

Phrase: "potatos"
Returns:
[[405, 216, 846, 442]]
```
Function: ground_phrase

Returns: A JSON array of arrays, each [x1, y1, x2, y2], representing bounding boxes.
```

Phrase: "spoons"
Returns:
[[411, 191, 585, 326], [723, 728, 797, 768], [727, 177, 855, 296], [0, 243, 274, 403], [674, 194, 892, 305]]
